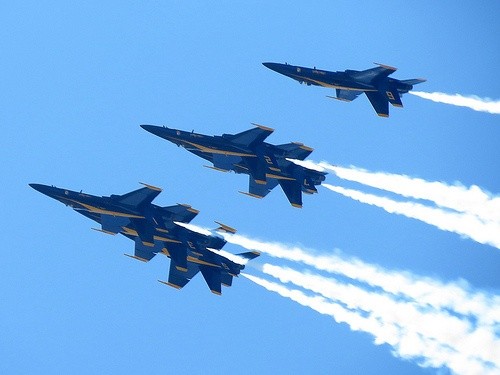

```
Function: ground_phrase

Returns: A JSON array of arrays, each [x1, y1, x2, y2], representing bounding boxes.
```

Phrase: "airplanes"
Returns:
[[259, 61, 426, 118], [27, 181, 262, 295], [137, 122, 331, 210]]
